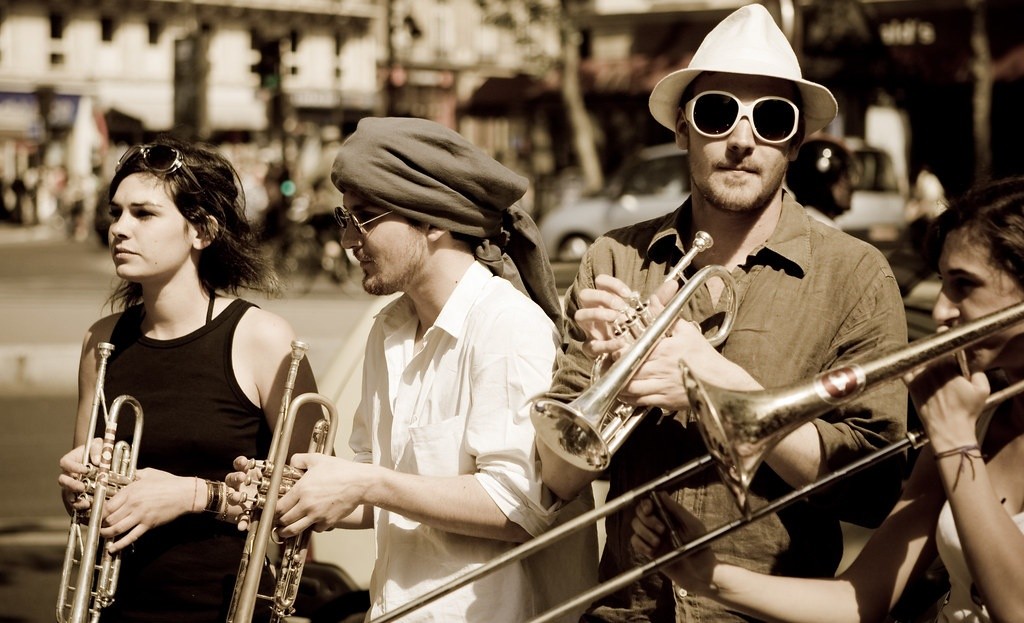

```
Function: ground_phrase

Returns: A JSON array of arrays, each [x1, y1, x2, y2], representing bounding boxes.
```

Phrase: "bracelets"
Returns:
[[190, 475, 229, 521]]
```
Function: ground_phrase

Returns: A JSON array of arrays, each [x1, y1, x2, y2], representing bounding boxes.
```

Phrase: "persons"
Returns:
[[235, 113, 607, 622], [785, 140, 861, 230], [628, 178, 1024, 623], [535, 0, 916, 623], [54, 137, 349, 623]]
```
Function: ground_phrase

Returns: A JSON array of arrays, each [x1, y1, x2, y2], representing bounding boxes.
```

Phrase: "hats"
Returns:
[[331, 116, 562, 322], [649, 3, 838, 138]]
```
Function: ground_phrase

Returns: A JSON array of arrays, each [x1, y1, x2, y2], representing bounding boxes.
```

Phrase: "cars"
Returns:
[[536, 134, 901, 269]]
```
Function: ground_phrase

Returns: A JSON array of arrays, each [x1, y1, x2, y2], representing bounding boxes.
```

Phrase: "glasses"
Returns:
[[115, 145, 207, 196], [684, 90, 800, 144], [334, 209, 396, 235]]
